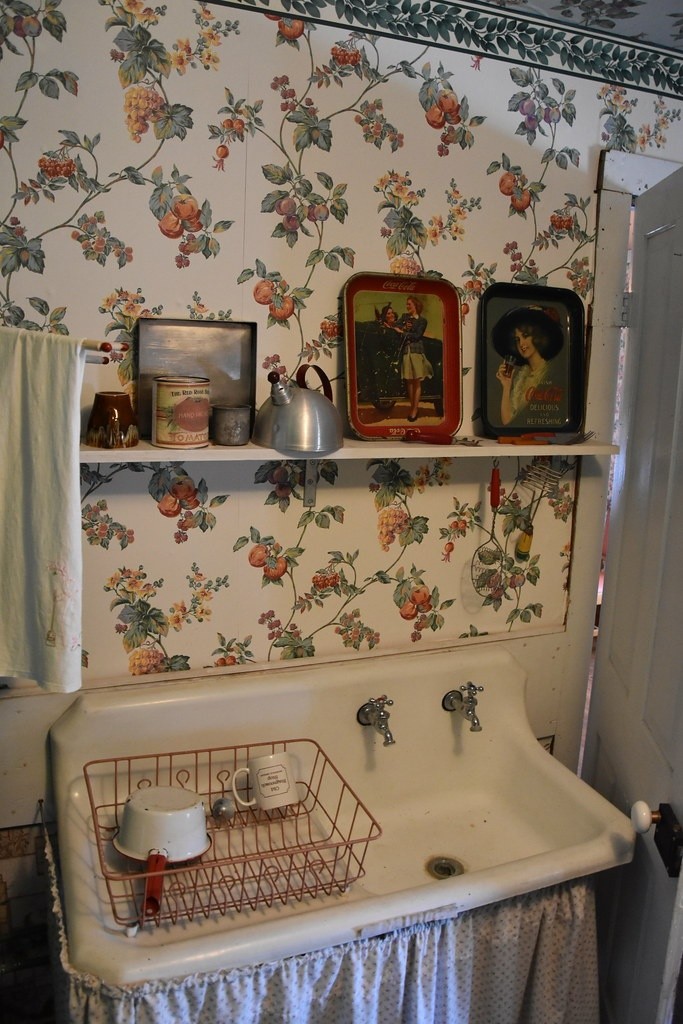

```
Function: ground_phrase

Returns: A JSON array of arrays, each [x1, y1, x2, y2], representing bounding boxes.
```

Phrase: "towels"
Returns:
[[0, 326, 88, 694]]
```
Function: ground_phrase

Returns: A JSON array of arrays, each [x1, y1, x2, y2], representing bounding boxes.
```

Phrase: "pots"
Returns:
[[112, 786, 213, 917]]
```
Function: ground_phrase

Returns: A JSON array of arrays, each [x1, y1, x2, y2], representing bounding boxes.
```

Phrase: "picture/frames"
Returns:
[[135, 317, 258, 440], [344, 271, 463, 441], [476, 281, 585, 437]]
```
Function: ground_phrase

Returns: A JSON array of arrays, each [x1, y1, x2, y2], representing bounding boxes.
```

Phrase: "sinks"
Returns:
[[46, 645, 635, 985]]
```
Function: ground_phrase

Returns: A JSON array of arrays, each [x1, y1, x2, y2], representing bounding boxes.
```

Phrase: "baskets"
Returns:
[[83, 737, 383, 928]]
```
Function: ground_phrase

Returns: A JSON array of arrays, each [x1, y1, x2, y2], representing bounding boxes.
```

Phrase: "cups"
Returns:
[[211, 403, 251, 446], [84, 391, 139, 449], [232, 752, 299, 811]]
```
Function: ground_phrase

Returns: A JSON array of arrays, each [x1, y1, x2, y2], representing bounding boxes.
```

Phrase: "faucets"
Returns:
[[356, 696, 395, 747], [442, 681, 484, 732]]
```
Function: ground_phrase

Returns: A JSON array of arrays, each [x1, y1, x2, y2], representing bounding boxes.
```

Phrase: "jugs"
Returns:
[[250, 364, 344, 452]]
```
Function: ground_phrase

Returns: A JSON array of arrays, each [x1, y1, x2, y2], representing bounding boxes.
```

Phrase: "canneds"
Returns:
[[151, 376, 211, 450]]
